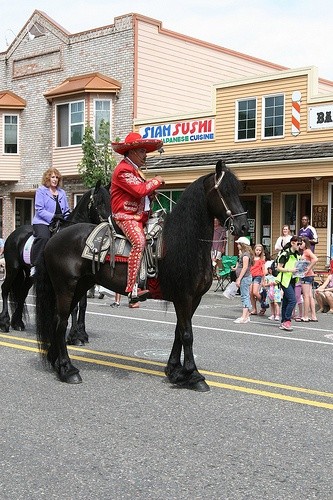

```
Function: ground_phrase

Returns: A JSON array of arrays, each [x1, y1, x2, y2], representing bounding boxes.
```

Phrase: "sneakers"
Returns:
[[278, 320, 294, 331]]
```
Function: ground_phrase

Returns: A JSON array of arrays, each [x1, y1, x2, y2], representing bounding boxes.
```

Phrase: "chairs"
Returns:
[[314, 259, 333, 283], [213, 255, 238, 292]]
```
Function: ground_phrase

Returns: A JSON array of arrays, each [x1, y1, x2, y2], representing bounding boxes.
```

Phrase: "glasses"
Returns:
[[296, 242, 302, 246]]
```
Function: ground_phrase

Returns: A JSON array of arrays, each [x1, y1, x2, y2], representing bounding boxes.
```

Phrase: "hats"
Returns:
[[110, 131, 163, 155], [234, 236, 251, 246]]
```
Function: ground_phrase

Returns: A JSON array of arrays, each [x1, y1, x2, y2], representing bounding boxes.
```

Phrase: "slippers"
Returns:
[[295, 318, 310, 322], [309, 318, 319, 322]]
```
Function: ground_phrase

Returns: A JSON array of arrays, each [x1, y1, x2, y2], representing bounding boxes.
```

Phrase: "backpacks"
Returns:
[[271, 250, 289, 277]]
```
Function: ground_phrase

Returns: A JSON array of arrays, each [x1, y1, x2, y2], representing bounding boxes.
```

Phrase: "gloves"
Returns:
[[53, 213, 66, 221]]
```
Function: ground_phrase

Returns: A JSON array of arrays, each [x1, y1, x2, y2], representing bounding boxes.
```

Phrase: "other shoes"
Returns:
[[252, 309, 266, 316], [233, 316, 251, 324], [110, 301, 121, 309], [274, 315, 280, 321], [98, 293, 104, 299], [128, 287, 151, 303], [268, 315, 274, 320], [87, 293, 95, 298]]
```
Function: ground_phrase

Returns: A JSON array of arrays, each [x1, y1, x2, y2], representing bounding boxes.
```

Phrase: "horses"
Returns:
[[0, 179, 111, 345], [32, 159, 250, 392]]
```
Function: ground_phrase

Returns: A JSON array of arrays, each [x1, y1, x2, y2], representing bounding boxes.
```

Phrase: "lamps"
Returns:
[[27, 22, 50, 41], [4, 28, 21, 47], [158, 147, 165, 156]]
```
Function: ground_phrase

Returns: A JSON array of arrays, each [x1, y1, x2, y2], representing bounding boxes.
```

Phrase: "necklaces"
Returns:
[[50, 189, 57, 198]]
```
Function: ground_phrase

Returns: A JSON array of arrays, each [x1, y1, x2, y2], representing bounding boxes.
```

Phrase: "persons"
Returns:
[[110, 293, 139, 308], [298, 215, 318, 253], [0, 238, 6, 280], [210, 218, 226, 280], [275, 224, 293, 257], [277, 236, 308, 331], [230, 237, 266, 324], [87, 285, 105, 299], [298, 237, 318, 322], [291, 282, 303, 320], [313, 260, 333, 314], [262, 261, 281, 321], [111, 132, 165, 304], [29, 168, 72, 278]]
